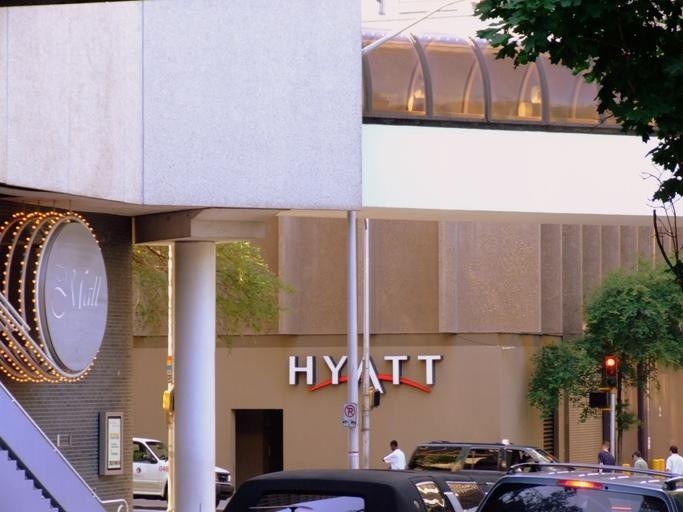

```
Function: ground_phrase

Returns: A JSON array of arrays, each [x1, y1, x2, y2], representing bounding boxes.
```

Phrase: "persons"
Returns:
[[381, 440, 407, 470], [665, 445, 683, 487], [632, 450, 648, 477], [597, 441, 615, 474]]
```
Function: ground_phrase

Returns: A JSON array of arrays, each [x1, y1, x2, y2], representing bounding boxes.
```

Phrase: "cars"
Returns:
[[131, 436, 234, 507]]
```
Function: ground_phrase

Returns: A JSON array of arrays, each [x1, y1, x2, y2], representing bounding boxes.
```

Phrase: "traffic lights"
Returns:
[[604, 354, 618, 392], [589, 391, 609, 408]]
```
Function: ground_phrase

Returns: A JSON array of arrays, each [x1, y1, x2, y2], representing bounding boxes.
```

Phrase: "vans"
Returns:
[[404, 439, 577, 482]]
